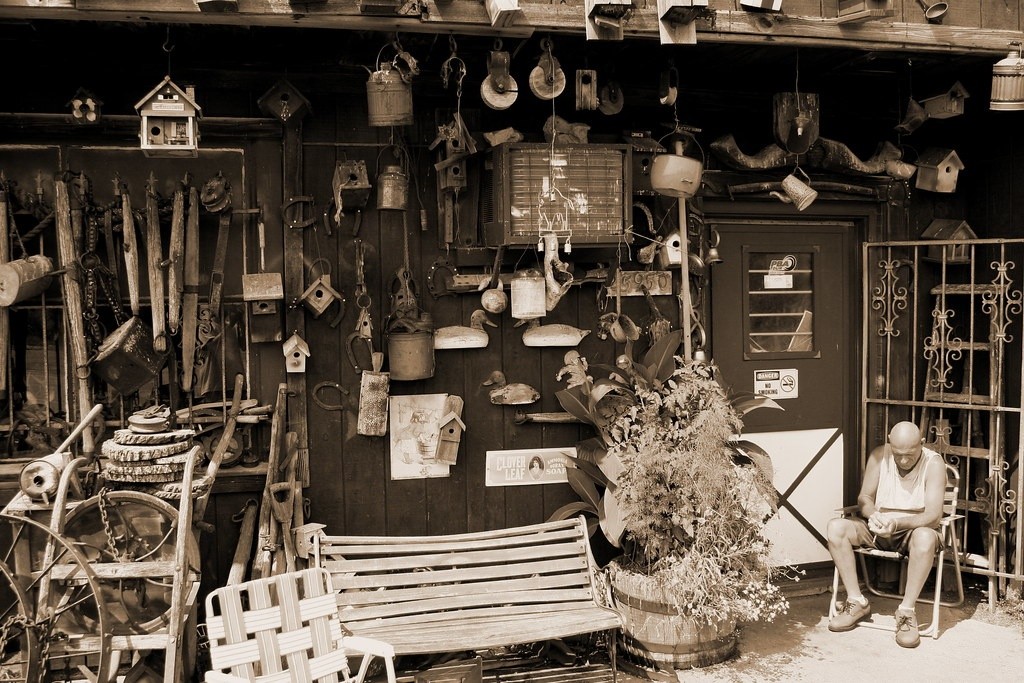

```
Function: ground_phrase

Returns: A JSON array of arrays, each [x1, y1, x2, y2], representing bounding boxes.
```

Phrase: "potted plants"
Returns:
[[546, 329, 807, 670]]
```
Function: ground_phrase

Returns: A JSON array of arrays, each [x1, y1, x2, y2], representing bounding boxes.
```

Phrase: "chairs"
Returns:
[[205, 567, 397, 683], [828, 464, 966, 640]]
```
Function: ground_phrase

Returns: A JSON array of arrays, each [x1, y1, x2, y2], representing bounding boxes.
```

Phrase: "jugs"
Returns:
[[769, 166, 818, 211]]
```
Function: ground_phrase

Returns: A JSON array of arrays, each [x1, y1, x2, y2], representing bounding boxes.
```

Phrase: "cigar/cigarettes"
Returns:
[[872, 525, 880, 543]]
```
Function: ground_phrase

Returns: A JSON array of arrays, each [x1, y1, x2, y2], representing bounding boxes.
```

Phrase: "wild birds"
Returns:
[[513, 318, 592, 347], [430, 309, 498, 349], [482, 371, 540, 405]]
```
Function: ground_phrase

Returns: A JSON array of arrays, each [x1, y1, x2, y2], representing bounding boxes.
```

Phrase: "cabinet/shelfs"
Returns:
[[7, 461, 269, 683]]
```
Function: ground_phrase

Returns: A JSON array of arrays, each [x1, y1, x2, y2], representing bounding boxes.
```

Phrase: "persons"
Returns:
[[827, 421, 946, 648], [529, 456, 543, 479]]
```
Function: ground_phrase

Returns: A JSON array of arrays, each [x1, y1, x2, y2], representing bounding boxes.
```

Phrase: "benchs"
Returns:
[[314, 515, 627, 683]]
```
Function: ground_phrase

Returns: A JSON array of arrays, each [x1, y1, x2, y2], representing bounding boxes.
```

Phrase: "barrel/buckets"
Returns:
[[651, 128, 704, 197], [384, 302, 435, 381], [366, 42, 415, 127], [510, 238, 547, 319], [375, 144, 411, 210], [989, 41, 1024, 111]]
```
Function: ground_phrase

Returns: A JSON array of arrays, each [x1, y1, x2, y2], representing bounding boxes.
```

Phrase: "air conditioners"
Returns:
[[478, 149, 623, 249]]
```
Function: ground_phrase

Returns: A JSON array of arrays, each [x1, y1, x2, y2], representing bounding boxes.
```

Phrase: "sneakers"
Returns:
[[828, 597, 871, 632], [895, 608, 920, 647]]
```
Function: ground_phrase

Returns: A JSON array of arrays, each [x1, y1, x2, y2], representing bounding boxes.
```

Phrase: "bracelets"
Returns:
[[893, 517, 898, 534]]
